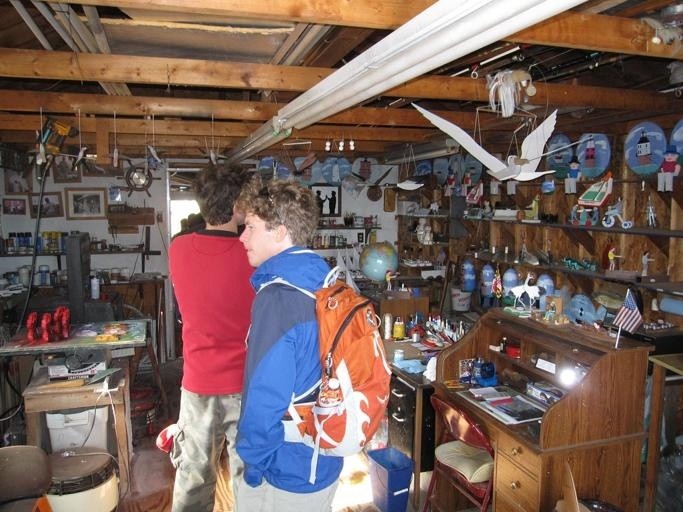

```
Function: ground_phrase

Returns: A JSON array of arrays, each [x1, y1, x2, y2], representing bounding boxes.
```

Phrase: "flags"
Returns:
[[612, 289, 644, 335]]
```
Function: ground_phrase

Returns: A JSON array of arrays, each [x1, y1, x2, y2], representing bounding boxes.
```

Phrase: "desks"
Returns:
[[339, 268, 432, 291], [643, 353, 683, 512], [384, 332, 452, 510], [0, 320, 167, 436]]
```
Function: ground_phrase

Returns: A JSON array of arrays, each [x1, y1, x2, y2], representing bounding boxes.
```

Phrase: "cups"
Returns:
[[328, 219, 336, 228], [371, 216, 376, 227], [393, 349, 404, 362], [353, 216, 364, 229], [412, 288, 420, 297], [343, 216, 353, 227], [411, 332, 420, 343]]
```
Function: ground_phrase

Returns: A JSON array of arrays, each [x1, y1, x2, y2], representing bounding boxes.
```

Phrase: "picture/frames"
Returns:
[[2, 154, 107, 220], [308, 182, 342, 217]]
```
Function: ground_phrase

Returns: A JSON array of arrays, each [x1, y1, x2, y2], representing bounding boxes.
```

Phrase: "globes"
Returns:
[[359, 240, 398, 294]]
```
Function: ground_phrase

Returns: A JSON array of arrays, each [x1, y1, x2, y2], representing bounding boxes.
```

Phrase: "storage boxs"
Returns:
[[22, 367, 131, 499]]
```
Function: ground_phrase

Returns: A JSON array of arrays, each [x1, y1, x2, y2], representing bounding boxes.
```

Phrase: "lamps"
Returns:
[[223, 112, 294, 164], [275, 0, 590, 134]]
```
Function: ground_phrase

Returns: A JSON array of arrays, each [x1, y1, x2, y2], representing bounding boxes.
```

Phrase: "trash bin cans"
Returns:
[[366, 447, 415, 512]]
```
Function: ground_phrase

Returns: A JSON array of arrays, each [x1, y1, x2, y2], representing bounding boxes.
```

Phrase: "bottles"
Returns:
[[92, 235, 97, 249], [498, 336, 507, 354], [324, 255, 336, 268], [7, 230, 82, 253], [18, 264, 129, 287], [312, 231, 344, 249], [392, 316, 405, 340]]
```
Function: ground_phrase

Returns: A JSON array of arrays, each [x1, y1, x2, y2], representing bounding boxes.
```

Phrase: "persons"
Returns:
[[326, 191, 336, 215], [560, 154, 584, 195], [315, 190, 327, 215], [232, 173, 343, 511], [608, 247, 622, 271], [601, 195, 633, 229], [385, 270, 400, 291], [168, 162, 258, 512], [42, 196, 57, 215], [442, 170, 472, 198], [641, 250, 655, 277], [652, 143, 681, 193]]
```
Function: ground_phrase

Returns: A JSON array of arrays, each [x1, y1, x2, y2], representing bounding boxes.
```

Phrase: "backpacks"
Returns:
[[245, 266, 392, 457]]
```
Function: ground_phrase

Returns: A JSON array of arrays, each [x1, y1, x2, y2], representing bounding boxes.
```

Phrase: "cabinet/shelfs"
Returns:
[[12, 276, 168, 364], [361, 290, 429, 334], [309, 225, 382, 249], [434, 307, 655, 512], [396, 116, 683, 352]]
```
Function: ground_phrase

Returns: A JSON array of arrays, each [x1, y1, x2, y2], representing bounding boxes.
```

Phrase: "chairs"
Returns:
[[0, 445, 51, 512], [423, 395, 494, 512]]
